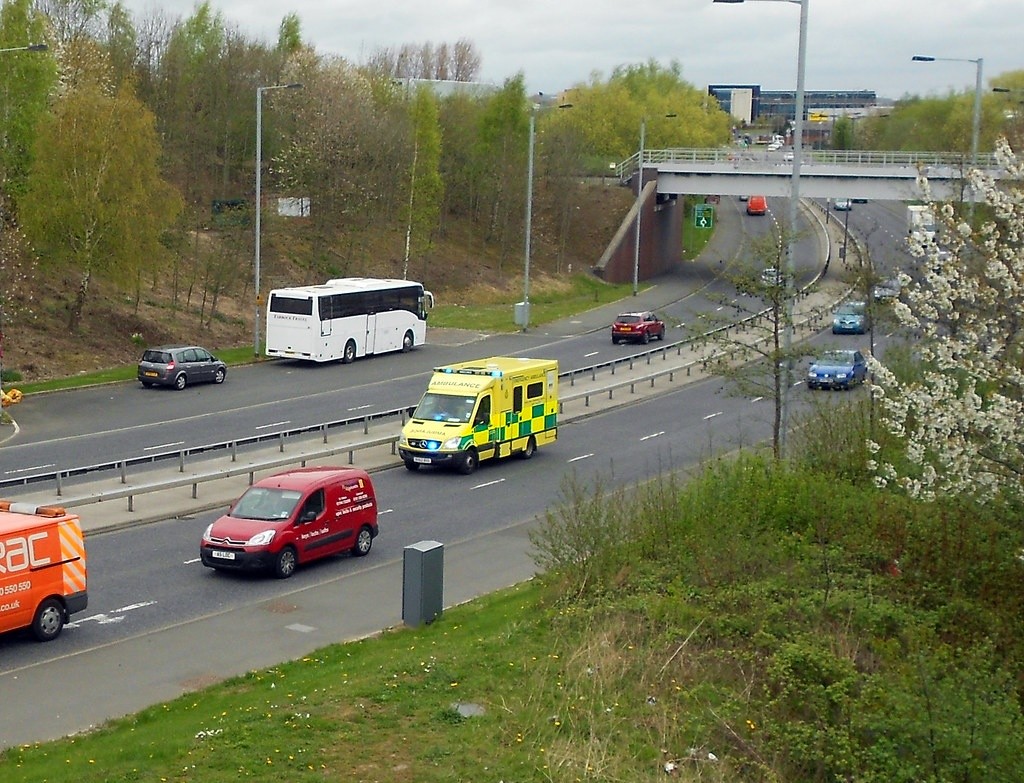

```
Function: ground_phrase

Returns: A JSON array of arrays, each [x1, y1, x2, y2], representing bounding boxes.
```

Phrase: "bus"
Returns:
[[266, 277, 434, 364]]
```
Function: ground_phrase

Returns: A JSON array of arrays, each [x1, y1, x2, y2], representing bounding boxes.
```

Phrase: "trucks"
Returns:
[[906, 204, 939, 245]]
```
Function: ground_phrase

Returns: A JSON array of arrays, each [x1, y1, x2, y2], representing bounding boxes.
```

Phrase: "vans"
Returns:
[[746, 196, 768, 216], [0, 500, 89, 643]]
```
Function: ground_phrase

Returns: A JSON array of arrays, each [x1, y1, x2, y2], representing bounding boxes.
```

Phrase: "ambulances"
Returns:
[[397, 356, 559, 476]]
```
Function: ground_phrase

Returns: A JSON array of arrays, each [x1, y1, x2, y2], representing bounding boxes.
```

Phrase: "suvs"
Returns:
[[611, 311, 666, 345]]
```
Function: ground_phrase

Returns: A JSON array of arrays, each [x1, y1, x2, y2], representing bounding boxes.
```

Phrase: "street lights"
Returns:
[[253, 83, 304, 359], [521, 103, 577, 333], [913, 57, 983, 367], [711, 1, 810, 465], [632, 112, 679, 298]]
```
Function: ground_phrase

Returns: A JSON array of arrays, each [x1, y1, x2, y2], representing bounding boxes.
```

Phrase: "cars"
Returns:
[[739, 196, 749, 201], [834, 198, 853, 211], [200, 464, 380, 579], [833, 300, 872, 336], [854, 198, 870, 203], [138, 343, 228, 391], [762, 269, 784, 290], [807, 349, 867, 391], [876, 278, 903, 301], [739, 132, 794, 161]]
